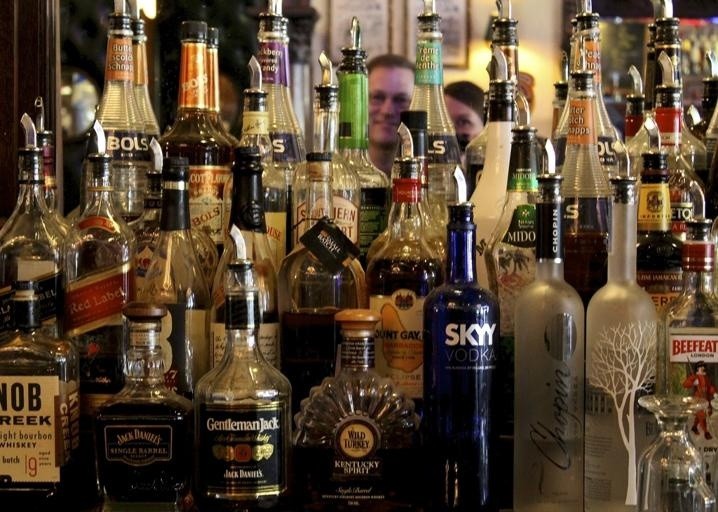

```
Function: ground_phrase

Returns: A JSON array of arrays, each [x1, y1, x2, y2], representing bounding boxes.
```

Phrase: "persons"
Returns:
[[442, 81, 487, 145], [362, 54, 416, 177], [683, 361, 714, 440]]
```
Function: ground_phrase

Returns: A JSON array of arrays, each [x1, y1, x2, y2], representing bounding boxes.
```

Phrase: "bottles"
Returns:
[[585, 174, 658, 510], [465, 79, 542, 222], [66, 156, 137, 396], [159, 21, 232, 208], [31, 131, 71, 238], [130, 170, 164, 250], [140, 158, 212, 400], [212, 147, 283, 372], [689, 78, 717, 143], [635, 86, 706, 238], [622, 91, 646, 146], [93, 14, 148, 166], [0, 147, 68, 338], [513, 175, 587, 509], [399, 16, 467, 193], [280, 148, 369, 388], [424, 201, 502, 512], [472, 17, 531, 130], [118, 21, 160, 143], [0, 280, 79, 503], [37, 68, 107, 179], [638, 23, 664, 122], [484, 129, 558, 438], [293, 306, 421, 512], [367, 158, 444, 416], [656, 219, 717, 491], [92, 301, 196, 512], [549, 68, 620, 300], [224, 87, 288, 268], [186, 29, 242, 146], [292, 88, 362, 246], [631, 15, 706, 179], [273, 18, 309, 158], [387, 111, 427, 222], [257, 12, 301, 177], [195, 260, 293, 508], [631, 151, 684, 301], [555, 13, 630, 180], [554, 19, 573, 139]]
